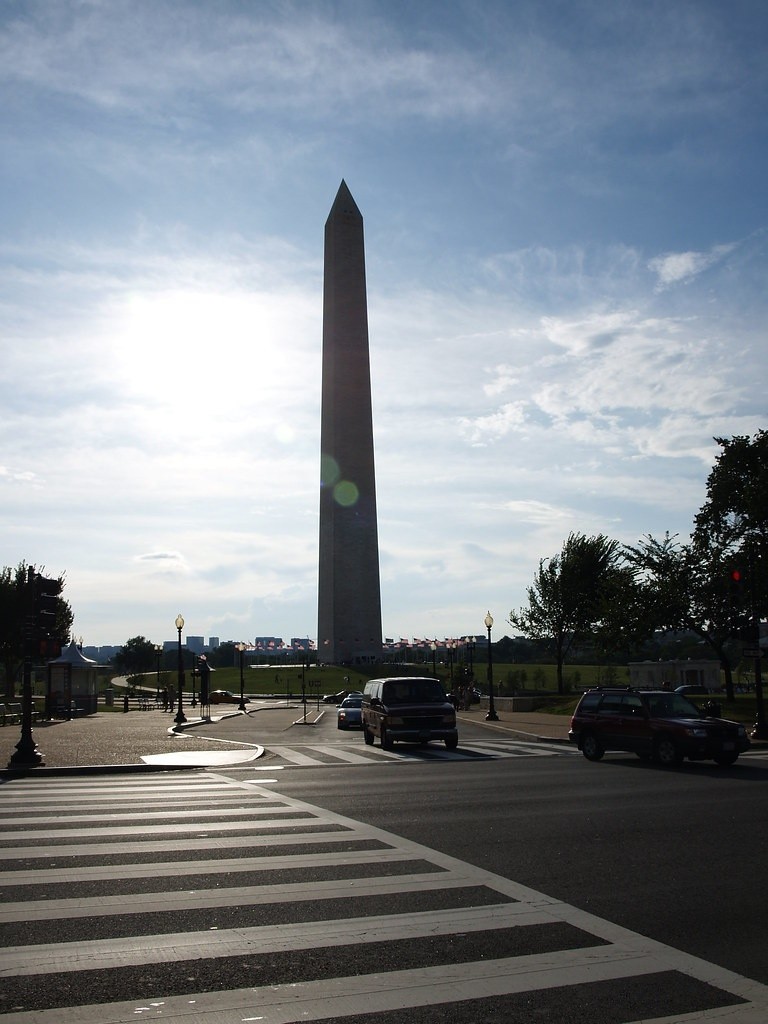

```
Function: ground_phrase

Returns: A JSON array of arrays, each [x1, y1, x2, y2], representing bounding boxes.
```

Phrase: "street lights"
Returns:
[[466, 637, 477, 673], [235, 642, 247, 710], [173, 614, 187, 723], [79, 636, 84, 653], [429, 641, 437, 674], [484, 611, 499, 720], [446, 642, 456, 678], [155, 645, 163, 699]]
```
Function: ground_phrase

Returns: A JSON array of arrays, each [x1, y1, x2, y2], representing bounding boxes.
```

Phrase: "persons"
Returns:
[[161, 684, 176, 712], [454, 681, 474, 712]]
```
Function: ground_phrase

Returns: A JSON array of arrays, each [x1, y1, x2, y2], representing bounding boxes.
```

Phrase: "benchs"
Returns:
[[139, 699, 156, 711], [0, 701, 43, 725]]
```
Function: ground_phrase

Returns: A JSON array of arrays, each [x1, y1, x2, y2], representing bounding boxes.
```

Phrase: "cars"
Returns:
[[323, 689, 363, 702], [674, 685, 709, 695], [459, 685, 487, 696], [210, 689, 250, 705], [348, 693, 364, 698], [191, 663, 217, 678], [335, 698, 362, 729], [446, 690, 486, 705]]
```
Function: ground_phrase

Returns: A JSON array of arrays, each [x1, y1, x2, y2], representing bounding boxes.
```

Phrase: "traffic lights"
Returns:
[[725, 561, 745, 605]]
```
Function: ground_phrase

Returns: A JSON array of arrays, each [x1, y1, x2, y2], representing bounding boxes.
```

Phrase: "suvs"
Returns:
[[568, 686, 751, 768], [361, 676, 458, 751]]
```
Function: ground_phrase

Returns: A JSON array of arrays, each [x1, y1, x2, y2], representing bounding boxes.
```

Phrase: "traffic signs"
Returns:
[[34, 576, 64, 596], [154, 650, 163, 656], [37, 596, 57, 612], [39, 613, 57, 627], [33, 640, 57, 657]]
